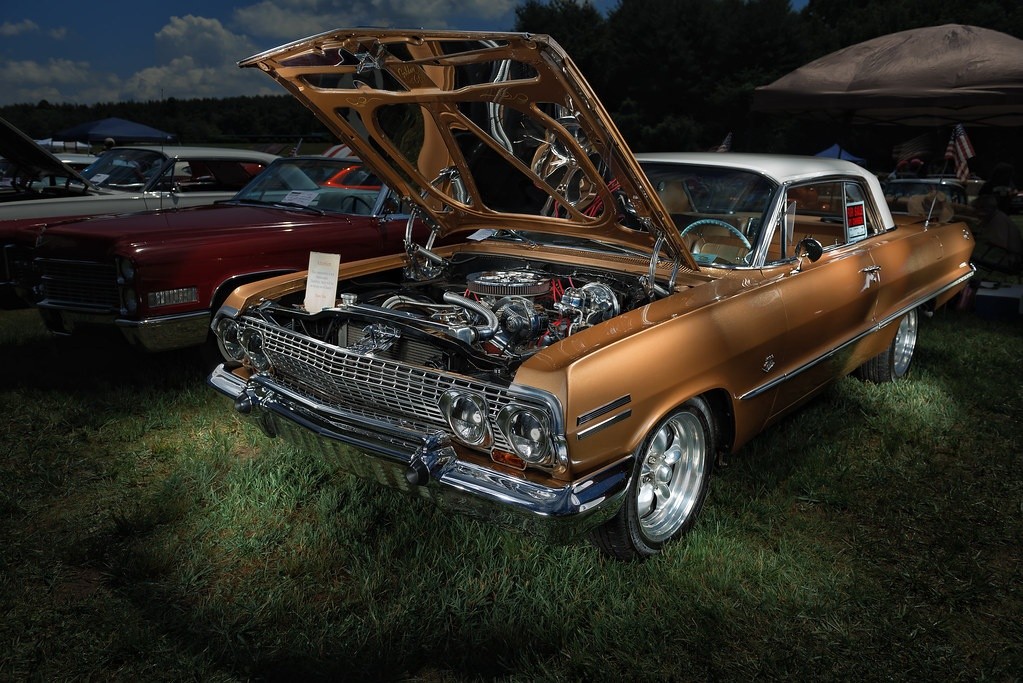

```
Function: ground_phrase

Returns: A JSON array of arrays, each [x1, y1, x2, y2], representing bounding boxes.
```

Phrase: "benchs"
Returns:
[[669, 212, 843, 265]]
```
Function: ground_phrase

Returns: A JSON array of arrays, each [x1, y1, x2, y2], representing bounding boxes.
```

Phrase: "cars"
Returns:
[[207, 26, 977, 560], [0, 117, 479, 364], [817, 172, 1023, 213]]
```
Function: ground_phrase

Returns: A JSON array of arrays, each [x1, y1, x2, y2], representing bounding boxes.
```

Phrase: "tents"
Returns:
[[51, 117, 178, 155], [754, 22, 1023, 128]]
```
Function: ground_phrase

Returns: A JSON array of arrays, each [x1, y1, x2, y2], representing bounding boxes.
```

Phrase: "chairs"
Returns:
[[691, 236, 752, 264]]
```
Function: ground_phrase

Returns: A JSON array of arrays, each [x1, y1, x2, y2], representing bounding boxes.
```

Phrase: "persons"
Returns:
[[98, 137, 114, 154]]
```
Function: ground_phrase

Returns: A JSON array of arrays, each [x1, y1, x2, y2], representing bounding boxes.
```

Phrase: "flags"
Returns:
[[718, 131, 734, 154], [947, 123, 976, 183]]
